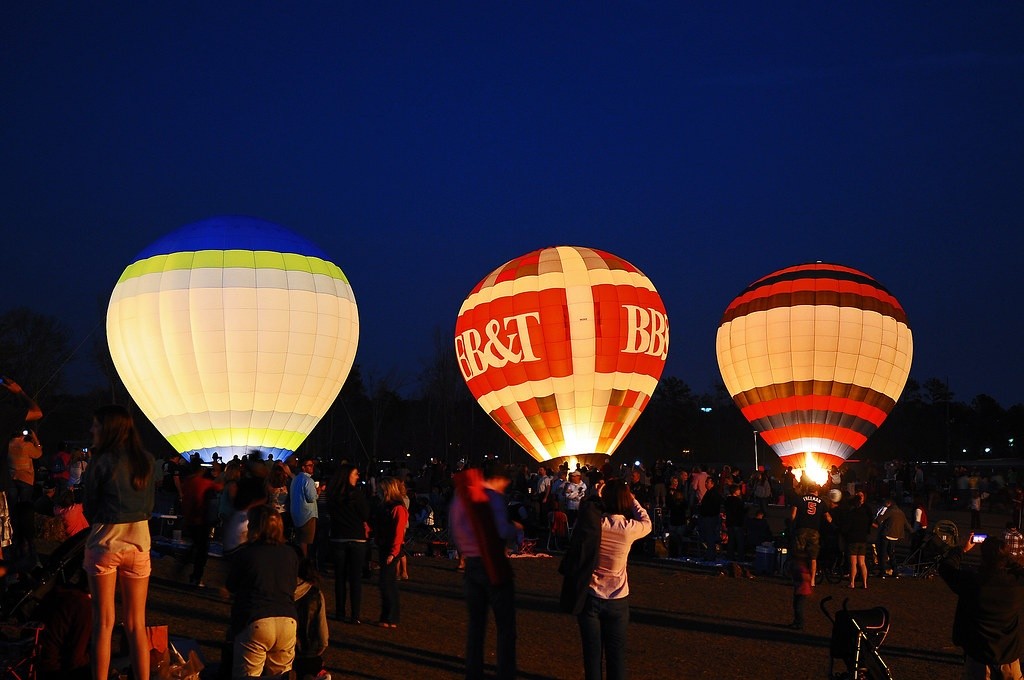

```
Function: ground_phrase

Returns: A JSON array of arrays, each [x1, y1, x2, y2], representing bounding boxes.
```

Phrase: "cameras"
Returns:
[[82, 448, 88, 453], [22, 430, 29, 436]]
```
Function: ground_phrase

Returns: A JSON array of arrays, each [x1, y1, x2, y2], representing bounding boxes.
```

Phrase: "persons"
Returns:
[[571, 478, 651, 680], [0, 377, 1024, 680]]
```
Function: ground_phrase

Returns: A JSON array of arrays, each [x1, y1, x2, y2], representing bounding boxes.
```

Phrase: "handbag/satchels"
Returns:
[[445, 574, 484, 601]]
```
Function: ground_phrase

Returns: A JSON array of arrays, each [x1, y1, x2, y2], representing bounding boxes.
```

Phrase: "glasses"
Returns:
[[305, 464, 315, 466], [1010, 529, 1018, 532]]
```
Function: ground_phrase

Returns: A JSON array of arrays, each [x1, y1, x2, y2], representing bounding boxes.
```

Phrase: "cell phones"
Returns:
[[972, 534, 988, 543]]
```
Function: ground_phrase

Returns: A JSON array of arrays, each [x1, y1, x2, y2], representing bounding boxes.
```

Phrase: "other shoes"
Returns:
[[378, 622, 397, 629], [847, 584, 854, 589], [328, 611, 346, 622], [861, 586, 867, 589], [792, 623, 803, 630], [457, 568, 466, 573], [891, 572, 897, 576], [350, 615, 359, 625], [396, 575, 408, 580], [189, 578, 205, 588], [878, 571, 885, 574]]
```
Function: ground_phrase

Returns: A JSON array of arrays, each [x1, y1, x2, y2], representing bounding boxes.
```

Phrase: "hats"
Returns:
[[723, 465, 731, 472], [572, 471, 581, 476]]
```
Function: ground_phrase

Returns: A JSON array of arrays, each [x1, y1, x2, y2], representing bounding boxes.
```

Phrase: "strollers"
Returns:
[[896, 520, 960, 581], [819, 594, 895, 679]]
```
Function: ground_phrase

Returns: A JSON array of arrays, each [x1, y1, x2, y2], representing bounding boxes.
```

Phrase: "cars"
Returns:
[[939, 475, 980, 512]]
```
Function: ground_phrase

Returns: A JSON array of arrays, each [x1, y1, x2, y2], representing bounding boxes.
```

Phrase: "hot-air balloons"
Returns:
[[716, 258, 913, 497], [452, 243, 671, 493], [105, 214, 363, 481]]
